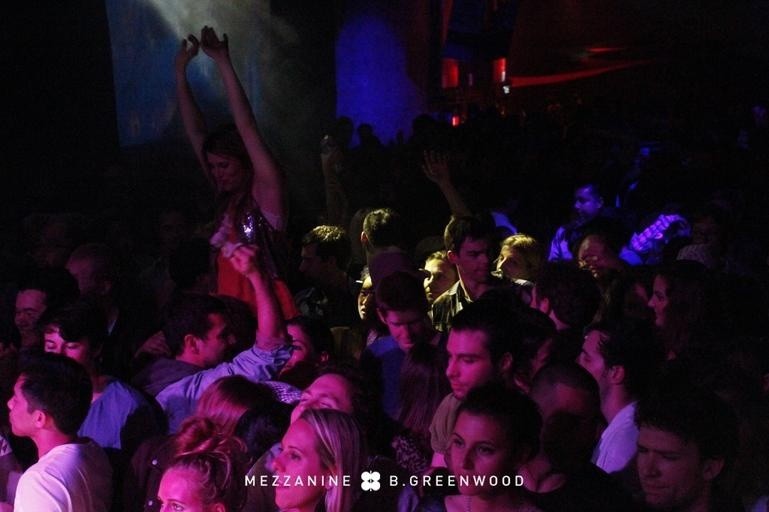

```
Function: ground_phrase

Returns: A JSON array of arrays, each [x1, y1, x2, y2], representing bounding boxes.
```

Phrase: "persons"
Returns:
[[170, 25, 286, 341], [0, 118, 769, 512]]
